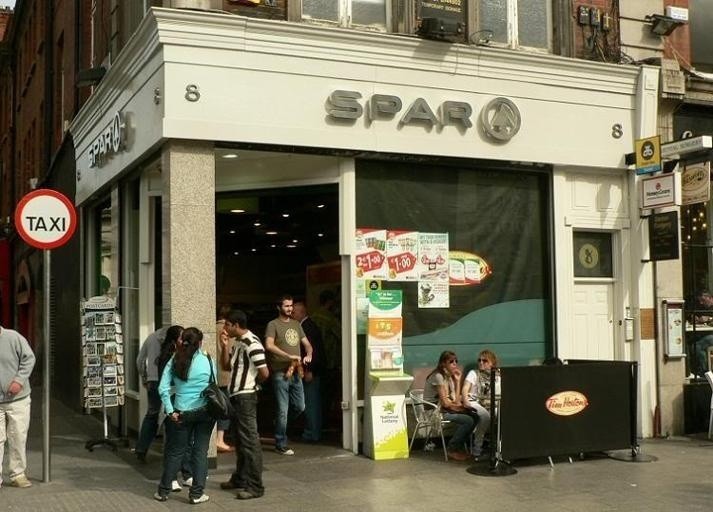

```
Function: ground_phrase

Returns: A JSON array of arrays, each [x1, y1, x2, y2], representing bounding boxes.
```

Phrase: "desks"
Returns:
[[684, 322, 713, 379]]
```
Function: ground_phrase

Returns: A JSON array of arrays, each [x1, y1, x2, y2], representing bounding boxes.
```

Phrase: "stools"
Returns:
[[702, 344, 712, 372]]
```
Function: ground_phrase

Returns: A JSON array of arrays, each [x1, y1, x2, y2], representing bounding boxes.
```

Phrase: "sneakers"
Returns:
[[12, 475, 32, 488], [275, 444, 295, 457], [220, 481, 236, 489], [152, 491, 169, 501], [235, 489, 254, 500], [171, 480, 182, 491], [447, 450, 472, 462], [189, 494, 211, 505]]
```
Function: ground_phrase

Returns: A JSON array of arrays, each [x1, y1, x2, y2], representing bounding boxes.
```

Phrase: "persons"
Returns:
[[423, 350, 474, 461], [689, 290, 712, 378], [0, 326, 36, 488], [462, 350, 497, 461]]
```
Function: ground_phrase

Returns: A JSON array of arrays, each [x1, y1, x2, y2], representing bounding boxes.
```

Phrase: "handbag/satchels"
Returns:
[[199, 354, 238, 421]]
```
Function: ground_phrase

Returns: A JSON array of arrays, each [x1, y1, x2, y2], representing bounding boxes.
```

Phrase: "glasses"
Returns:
[[449, 359, 458, 364], [477, 358, 489, 363]]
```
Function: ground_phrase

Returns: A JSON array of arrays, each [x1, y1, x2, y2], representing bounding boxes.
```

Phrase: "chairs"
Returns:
[[703, 370, 712, 440], [405, 386, 471, 463]]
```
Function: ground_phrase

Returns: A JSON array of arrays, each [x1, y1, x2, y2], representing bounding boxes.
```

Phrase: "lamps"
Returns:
[[73, 66, 107, 90], [643, 12, 680, 37]]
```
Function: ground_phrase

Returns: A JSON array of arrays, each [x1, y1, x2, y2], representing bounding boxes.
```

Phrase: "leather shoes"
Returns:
[[217, 444, 237, 453]]
